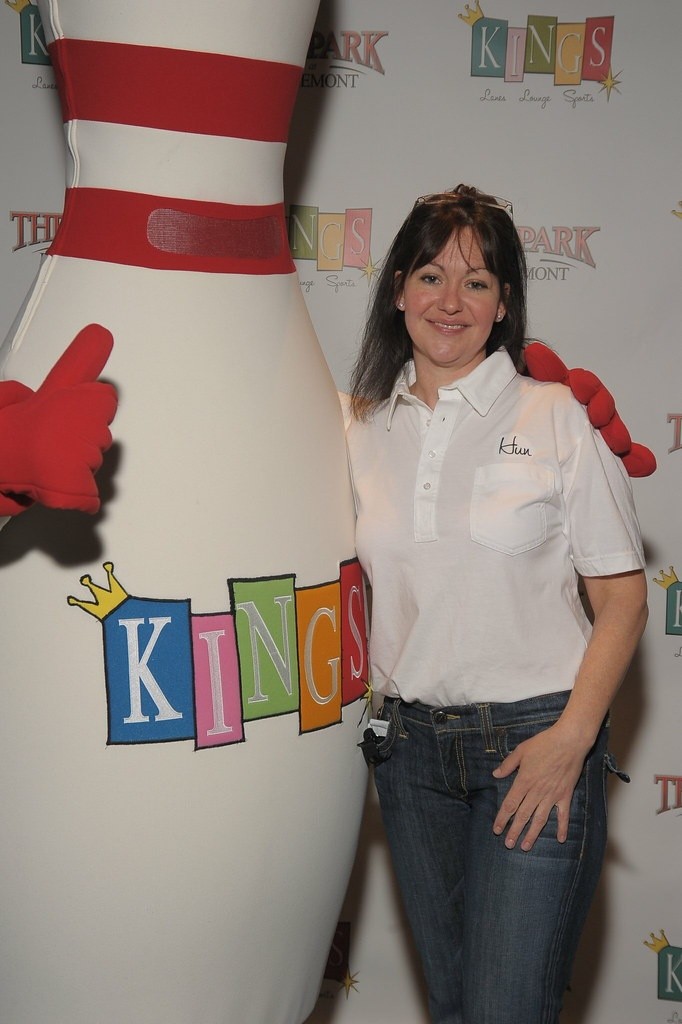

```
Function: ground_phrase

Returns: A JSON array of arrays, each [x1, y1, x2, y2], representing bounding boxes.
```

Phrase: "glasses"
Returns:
[[402, 190, 514, 240]]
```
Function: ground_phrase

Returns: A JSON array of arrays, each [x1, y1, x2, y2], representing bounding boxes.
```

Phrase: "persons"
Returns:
[[345, 182, 650, 1024]]
